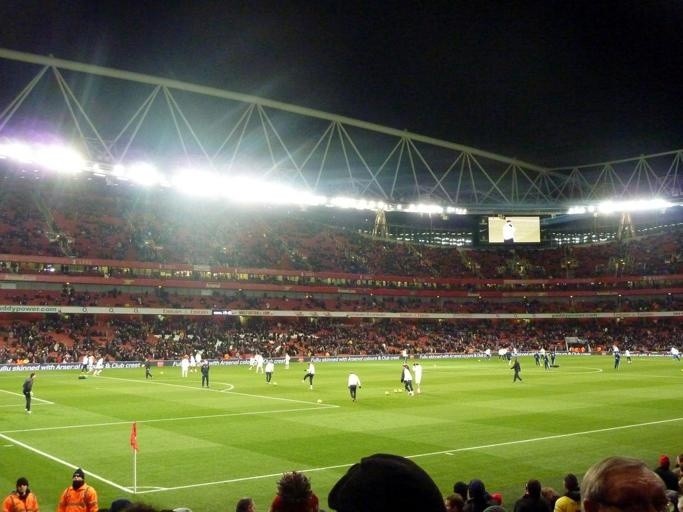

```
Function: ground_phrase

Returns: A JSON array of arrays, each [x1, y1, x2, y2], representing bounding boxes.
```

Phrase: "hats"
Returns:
[[469, 479, 484, 496], [658, 455, 669, 466], [490, 493, 502, 504], [268, 471, 318, 512]]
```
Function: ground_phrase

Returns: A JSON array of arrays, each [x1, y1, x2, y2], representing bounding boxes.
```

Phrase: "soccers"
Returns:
[[393, 389, 402, 393], [385, 391, 390, 396], [273, 381, 277, 386], [159, 371, 164, 375], [316, 399, 323, 404]]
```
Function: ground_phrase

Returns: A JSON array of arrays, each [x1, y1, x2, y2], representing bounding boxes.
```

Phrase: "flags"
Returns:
[[131, 423, 140, 451]]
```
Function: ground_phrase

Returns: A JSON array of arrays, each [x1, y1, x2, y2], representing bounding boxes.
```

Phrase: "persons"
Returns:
[[265, 362, 274, 384], [249, 354, 263, 374], [348, 371, 361, 403], [141, 358, 153, 379], [1, 454, 683, 512], [22, 373, 36, 413], [510, 358, 523, 383], [534, 351, 556, 372], [613, 351, 621, 370], [301, 362, 315, 391], [200, 362, 209, 388], [81, 354, 104, 376], [283, 353, 290, 369], [180, 354, 198, 378], [1, 190, 682, 364], [401, 362, 423, 396]]
